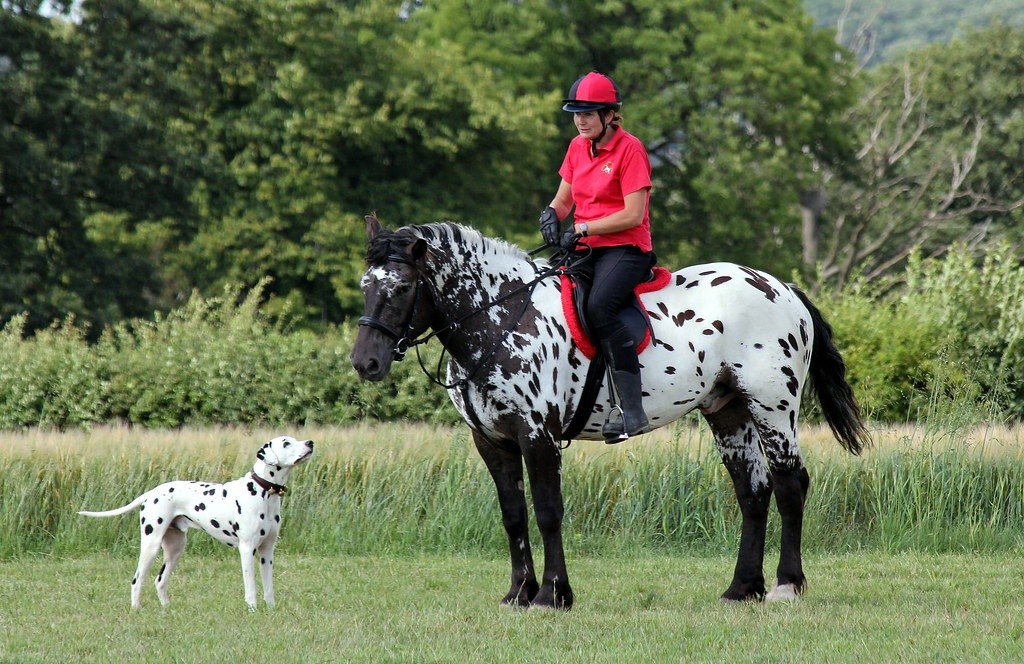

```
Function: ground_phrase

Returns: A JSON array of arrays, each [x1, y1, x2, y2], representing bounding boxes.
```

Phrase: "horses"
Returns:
[[348, 210, 875, 614]]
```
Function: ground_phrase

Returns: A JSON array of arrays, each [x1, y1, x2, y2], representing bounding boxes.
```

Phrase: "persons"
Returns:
[[538, 71, 658, 439]]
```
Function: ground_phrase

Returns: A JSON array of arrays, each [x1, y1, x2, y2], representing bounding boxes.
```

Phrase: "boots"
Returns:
[[603, 326, 650, 436]]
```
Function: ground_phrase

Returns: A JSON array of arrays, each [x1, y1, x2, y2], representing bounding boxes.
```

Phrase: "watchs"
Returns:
[[579, 222, 589, 238]]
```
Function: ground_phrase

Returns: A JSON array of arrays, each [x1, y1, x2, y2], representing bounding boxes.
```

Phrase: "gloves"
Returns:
[[539, 207, 560, 244], [560, 223, 576, 251]]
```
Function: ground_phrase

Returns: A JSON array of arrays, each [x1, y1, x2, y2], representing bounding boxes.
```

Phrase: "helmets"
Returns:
[[562, 71, 621, 113]]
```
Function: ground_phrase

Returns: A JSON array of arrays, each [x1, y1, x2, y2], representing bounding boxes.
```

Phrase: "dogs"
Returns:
[[79, 435, 314, 613]]
